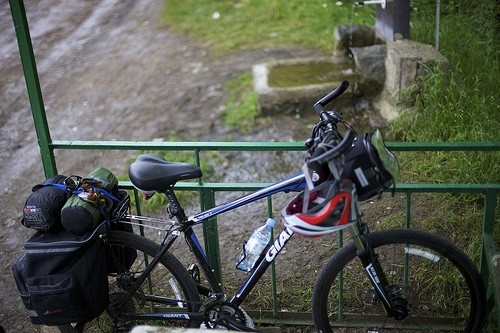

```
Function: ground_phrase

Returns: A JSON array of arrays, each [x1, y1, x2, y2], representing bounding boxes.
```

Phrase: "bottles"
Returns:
[[236, 218, 276, 274]]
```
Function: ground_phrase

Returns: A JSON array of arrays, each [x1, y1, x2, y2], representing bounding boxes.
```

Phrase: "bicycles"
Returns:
[[12, 80, 488, 332]]
